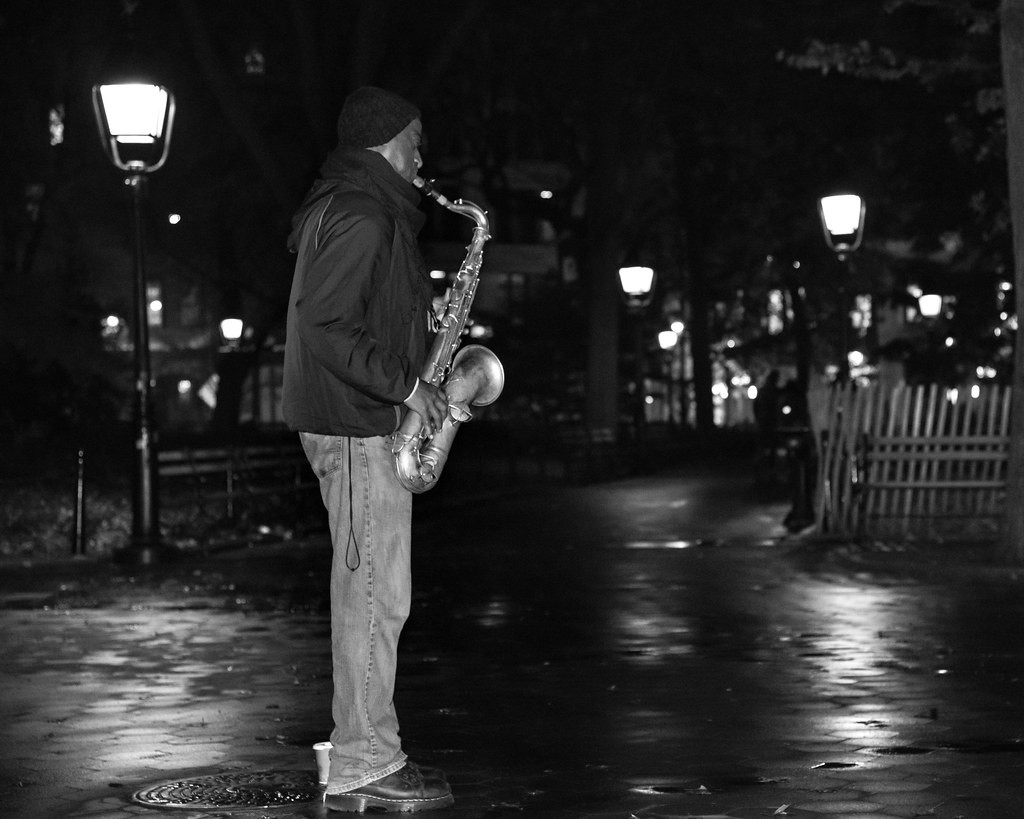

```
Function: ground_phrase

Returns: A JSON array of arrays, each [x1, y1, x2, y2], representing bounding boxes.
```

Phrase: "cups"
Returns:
[[314, 742, 332, 784]]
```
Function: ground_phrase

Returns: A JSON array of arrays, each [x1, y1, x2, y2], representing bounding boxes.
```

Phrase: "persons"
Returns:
[[281, 85, 456, 814]]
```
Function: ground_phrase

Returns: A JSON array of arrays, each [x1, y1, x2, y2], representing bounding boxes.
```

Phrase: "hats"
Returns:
[[337, 87, 421, 148]]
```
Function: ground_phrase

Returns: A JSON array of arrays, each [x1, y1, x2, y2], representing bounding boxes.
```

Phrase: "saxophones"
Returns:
[[392, 178, 505, 494]]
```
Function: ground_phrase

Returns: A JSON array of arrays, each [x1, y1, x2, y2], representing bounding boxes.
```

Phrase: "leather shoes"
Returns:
[[324, 760, 456, 815]]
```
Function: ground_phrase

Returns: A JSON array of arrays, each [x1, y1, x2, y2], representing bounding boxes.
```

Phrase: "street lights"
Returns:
[[811, 191, 869, 383], [616, 265, 657, 440], [85, 77, 181, 582]]
